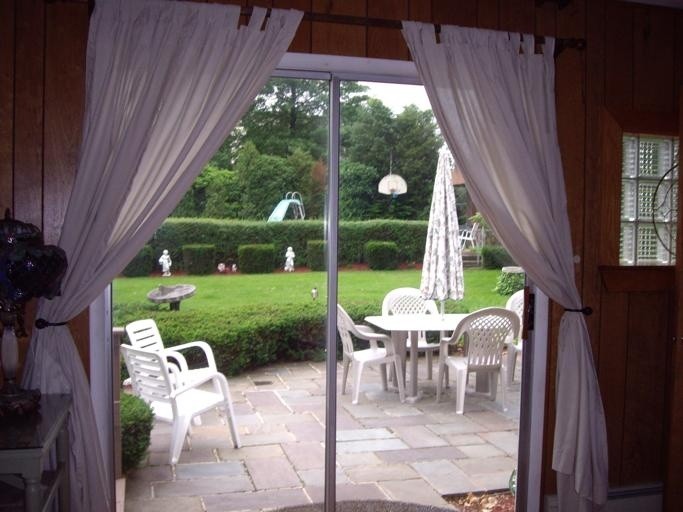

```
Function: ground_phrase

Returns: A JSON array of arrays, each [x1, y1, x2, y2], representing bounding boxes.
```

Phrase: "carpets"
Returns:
[[264, 500, 451, 511]]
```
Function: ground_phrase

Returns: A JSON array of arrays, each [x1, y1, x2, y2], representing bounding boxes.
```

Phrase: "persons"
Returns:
[[159, 248, 172, 277], [284, 245, 296, 272]]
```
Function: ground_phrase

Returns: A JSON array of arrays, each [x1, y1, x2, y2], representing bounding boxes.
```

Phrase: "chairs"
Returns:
[[119, 319, 243, 465], [336, 287, 524, 415]]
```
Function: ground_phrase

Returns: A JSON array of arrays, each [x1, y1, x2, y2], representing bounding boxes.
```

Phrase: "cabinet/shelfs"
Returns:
[[0, 393, 73, 512]]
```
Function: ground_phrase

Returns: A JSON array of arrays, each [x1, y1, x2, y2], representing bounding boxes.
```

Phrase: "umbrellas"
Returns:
[[417, 146, 464, 340]]
